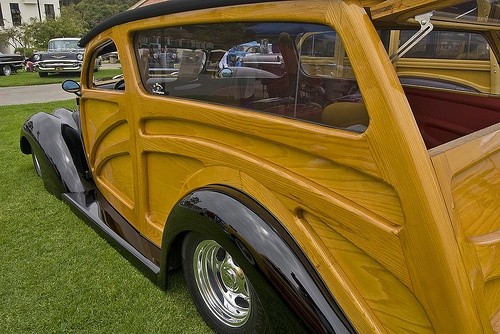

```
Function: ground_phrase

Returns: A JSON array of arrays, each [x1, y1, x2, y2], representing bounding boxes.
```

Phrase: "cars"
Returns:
[[32, 36, 103, 77], [0, 50, 26, 78], [227, 26, 499, 96], [18, 0, 499, 334]]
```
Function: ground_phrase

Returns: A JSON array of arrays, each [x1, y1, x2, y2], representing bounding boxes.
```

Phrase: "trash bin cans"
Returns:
[[27, 60, 33, 72], [109, 55, 116, 64]]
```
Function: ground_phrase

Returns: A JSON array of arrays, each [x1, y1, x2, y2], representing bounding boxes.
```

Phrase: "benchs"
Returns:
[[145, 78, 256, 100]]
